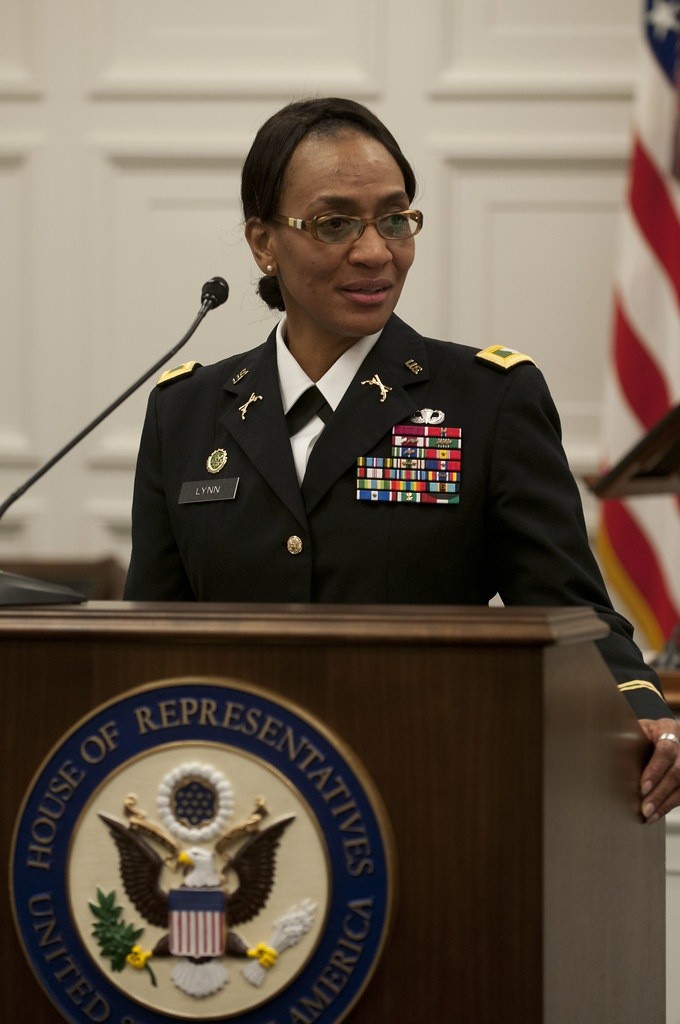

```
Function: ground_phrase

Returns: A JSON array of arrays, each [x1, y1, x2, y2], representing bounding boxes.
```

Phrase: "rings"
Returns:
[[657, 733, 680, 748]]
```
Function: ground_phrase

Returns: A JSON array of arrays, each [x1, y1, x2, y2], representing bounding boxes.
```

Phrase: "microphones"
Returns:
[[0, 278, 229, 603]]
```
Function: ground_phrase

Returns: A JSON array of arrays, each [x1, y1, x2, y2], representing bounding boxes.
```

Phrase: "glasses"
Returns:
[[271, 208, 423, 246]]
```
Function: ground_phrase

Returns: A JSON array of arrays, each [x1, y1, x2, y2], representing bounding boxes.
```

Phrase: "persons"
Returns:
[[114, 97, 680, 827]]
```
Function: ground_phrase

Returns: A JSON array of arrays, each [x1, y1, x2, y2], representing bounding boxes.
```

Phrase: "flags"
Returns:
[[589, 1, 679, 656]]
